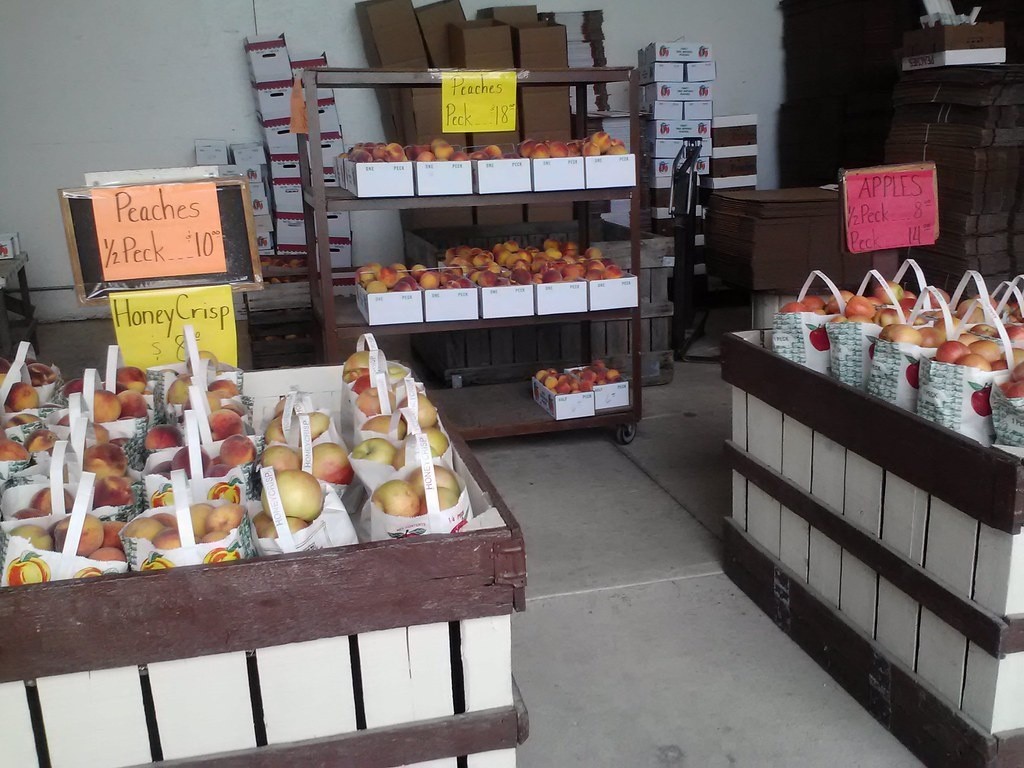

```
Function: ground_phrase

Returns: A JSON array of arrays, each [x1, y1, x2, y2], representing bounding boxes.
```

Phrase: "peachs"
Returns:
[[355, 237, 624, 292], [536, 360, 623, 395], [0, 350, 257, 561], [339, 131, 629, 163], [259, 256, 311, 341]]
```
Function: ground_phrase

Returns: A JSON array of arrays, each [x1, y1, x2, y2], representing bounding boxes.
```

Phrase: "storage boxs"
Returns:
[[194, 1, 757, 326], [902, 21, 1006, 71], [564, 367, 630, 409], [0, 232, 21, 259], [532, 373, 595, 420]]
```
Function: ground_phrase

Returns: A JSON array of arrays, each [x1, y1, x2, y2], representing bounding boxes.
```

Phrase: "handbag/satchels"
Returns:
[[889, 262, 1024, 348], [914, 298, 1016, 449], [0, 328, 473, 589], [989, 375, 1024, 448], [771, 270, 846, 377], [826, 269, 934, 390], [866, 285, 954, 413]]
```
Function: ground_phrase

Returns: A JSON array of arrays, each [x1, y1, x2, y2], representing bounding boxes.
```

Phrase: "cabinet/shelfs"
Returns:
[[294, 66, 642, 445]]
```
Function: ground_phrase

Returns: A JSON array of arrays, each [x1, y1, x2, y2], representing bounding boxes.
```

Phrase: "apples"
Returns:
[[778, 279, 1024, 400], [254, 350, 464, 539]]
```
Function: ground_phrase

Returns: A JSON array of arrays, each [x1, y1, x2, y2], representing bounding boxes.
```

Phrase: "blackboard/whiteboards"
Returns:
[[57, 174, 265, 308]]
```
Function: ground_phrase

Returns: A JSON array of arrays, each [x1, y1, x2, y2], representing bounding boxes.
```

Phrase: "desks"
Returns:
[[0, 251, 37, 360]]
